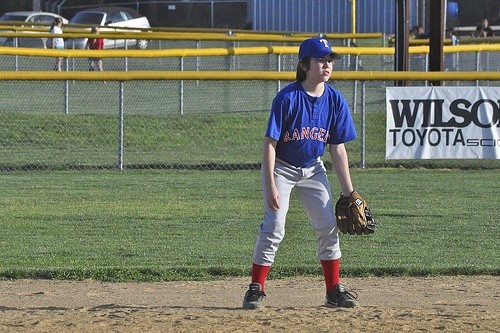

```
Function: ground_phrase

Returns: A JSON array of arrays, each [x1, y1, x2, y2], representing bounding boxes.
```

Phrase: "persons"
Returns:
[[85, 26, 106, 84], [413, 26, 429, 39], [50, 17, 64, 71], [350, 41, 364, 71], [474, 18, 494, 37], [444, 26, 460, 45], [243, 37, 376, 310]]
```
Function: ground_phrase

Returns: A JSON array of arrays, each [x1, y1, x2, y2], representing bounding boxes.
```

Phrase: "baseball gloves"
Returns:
[[335, 190, 375, 236]]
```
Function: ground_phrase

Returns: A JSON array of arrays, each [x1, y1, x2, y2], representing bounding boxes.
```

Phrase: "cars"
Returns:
[[1, 12, 69, 48], [69, 8, 151, 49]]
